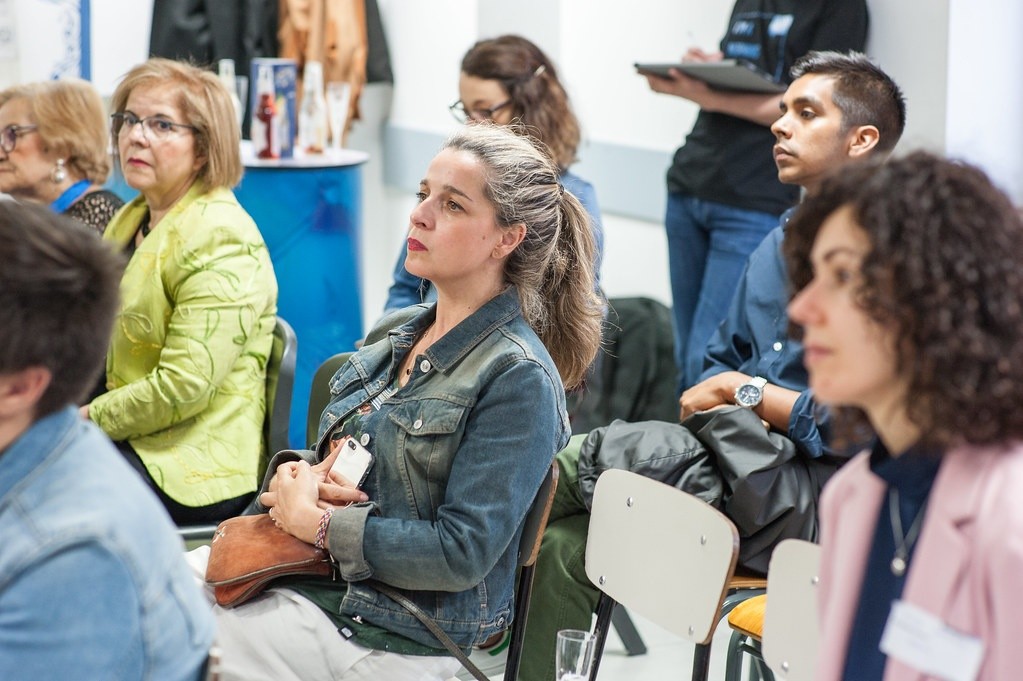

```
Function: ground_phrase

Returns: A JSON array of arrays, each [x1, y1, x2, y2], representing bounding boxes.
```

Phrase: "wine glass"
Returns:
[[324, 81, 353, 162]]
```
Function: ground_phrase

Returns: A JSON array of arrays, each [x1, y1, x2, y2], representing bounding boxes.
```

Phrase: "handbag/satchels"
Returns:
[[205, 513, 331, 609]]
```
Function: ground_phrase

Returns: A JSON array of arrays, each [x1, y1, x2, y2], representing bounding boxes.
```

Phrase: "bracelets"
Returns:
[[314, 507, 334, 549]]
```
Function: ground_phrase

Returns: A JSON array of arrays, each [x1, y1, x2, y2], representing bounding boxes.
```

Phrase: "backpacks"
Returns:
[[567, 297, 682, 436]]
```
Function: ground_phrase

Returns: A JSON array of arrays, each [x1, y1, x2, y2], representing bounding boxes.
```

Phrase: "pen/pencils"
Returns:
[[688, 31, 699, 49]]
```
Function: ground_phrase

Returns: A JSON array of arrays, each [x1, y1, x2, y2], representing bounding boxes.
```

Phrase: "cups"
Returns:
[[555, 628, 597, 681], [248, 56, 298, 160]]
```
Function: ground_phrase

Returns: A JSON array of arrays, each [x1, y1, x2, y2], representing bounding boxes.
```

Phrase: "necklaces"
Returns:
[[407, 321, 437, 375], [888, 487, 931, 578], [141, 210, 150, 237]]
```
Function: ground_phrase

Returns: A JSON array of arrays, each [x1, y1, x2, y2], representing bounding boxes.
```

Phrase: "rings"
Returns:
[[269, 506, 276, 522]]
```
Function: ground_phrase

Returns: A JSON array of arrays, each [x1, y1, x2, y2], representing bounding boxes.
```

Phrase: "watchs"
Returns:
[[733, 375, 768, 410]]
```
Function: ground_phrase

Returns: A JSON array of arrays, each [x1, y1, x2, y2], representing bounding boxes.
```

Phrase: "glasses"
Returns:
[[110, 113, 196, 142], [449, 100, 512, 126], [0, 123, 38, 152]]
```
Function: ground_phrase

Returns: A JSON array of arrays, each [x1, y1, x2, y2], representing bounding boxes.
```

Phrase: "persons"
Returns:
[[781, 152, 1023, 681], [518, 50, 904, 681], [184, 123, 608, 681], [0, 57, 279, 532], [0, 203, 214, 681], [380, 37, 603, 307], [665, 1, 870, 387]]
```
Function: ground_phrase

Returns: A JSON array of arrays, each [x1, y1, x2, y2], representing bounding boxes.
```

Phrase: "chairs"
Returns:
[[169, 313, 821, 681]]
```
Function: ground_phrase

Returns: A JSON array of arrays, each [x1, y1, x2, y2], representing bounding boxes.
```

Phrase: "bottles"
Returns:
[[299, 59, 329, 162], [216, 57, 243, 126]]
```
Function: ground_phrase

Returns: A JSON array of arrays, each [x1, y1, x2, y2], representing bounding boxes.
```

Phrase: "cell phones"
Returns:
[[325, 438, 375, 507]]
